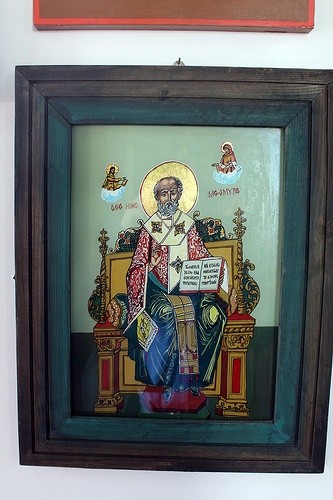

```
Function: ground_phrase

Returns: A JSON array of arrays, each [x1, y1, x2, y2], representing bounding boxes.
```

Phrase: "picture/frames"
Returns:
[[45, 94, 311, 449]]
